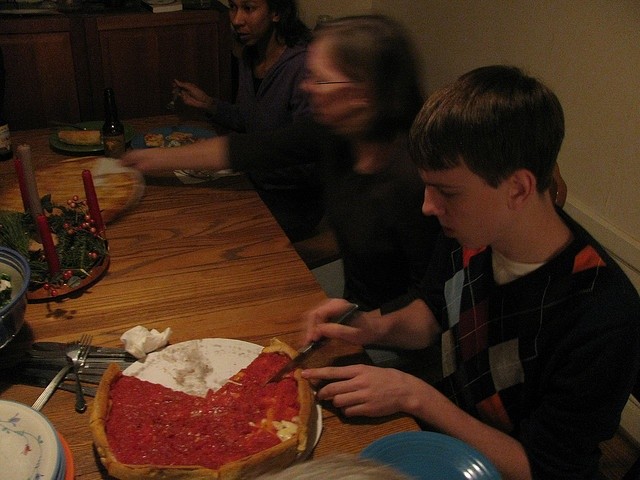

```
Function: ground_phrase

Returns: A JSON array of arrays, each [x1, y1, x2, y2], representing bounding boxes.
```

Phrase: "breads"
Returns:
[[57, 130, 102, 145]]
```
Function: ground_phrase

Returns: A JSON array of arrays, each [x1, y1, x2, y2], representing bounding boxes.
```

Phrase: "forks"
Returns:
[[31, 332, 93, 412], [66, 339, 86, 413]]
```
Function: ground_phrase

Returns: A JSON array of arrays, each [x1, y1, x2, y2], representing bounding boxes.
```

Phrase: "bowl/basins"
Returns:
[[0, 246, 31, 349]]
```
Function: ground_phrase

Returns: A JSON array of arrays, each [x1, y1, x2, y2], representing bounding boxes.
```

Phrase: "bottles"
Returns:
[[0, 115, 14, 160], [102, 86, 127, 160]]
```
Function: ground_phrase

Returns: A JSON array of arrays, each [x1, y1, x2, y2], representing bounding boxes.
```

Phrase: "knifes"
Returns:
[[267, 303, 363, 384]]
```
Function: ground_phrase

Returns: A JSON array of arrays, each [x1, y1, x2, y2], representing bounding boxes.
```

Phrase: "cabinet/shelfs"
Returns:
[[0, 18, 81, 131], [95, 9, 231, 120]]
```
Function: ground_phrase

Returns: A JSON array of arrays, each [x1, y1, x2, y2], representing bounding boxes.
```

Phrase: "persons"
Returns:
[[172, 0, 312, 132], [300, 65, 639, 480], [119, 15, 463, 314]]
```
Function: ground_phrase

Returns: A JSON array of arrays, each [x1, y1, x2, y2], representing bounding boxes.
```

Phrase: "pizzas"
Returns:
[[166, 130, 194, 145], [142, 132, 166, 149], [90, 337, 319, 480]]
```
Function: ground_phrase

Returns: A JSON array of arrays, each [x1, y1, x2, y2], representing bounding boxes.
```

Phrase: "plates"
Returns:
[[49, 120, 135, 153], [130, 127, 218, 150], [357, 430, 502, 479], [0, 400, 76, 480]]
